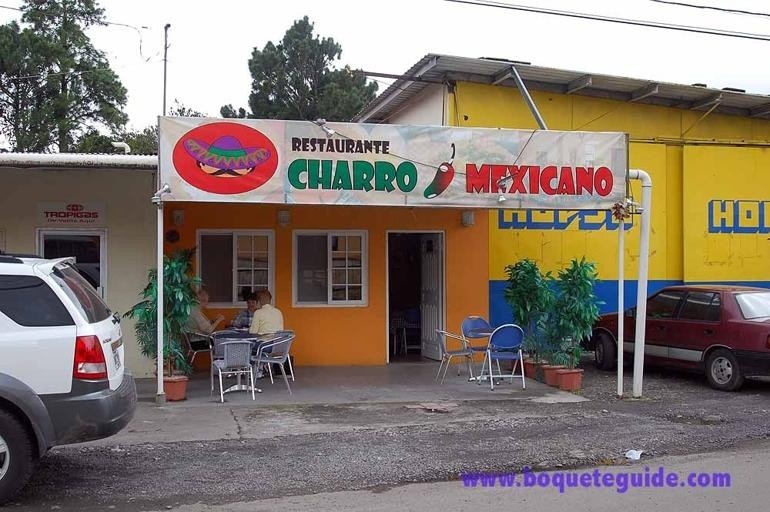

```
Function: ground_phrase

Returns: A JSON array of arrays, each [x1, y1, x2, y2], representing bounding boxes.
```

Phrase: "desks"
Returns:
[[468, 328, 496, 382]]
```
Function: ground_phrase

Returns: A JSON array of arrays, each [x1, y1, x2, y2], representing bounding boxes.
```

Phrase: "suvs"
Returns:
[[42, 236, 99, 280], [0, 251, 137, 506]]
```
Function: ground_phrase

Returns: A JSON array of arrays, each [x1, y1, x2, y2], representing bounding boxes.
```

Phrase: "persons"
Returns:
[[230, 292, 258, 328], [248, 289, 284, 380], [185, 285, 225, 351]]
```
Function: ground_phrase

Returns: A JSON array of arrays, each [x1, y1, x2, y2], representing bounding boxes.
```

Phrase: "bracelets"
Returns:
[[212, 320, 214, 323]]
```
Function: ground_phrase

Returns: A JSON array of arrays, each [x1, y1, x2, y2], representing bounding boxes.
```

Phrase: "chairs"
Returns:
[[391, 309, 421, 360], [457, 316, 503, 380], [478, 324, 526, 391], [434, 329, 473, 385], [178, 328, 296, 403]]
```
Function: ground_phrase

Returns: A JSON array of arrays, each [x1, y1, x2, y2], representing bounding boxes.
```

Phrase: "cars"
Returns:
[[581, 284, 770, 390]]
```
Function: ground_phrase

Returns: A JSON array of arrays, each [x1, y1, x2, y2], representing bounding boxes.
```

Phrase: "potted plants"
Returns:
[[122, 246, 203, 401], [502, 255, 606, 391]]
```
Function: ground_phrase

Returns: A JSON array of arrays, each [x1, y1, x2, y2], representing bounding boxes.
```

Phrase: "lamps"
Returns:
[[155, 183, 171, 203], [498, 188, 506, 202], [173, 209, 184, 225], [462, 212, 474, 226], [317, 119, 335, 138], [279, 210, 290, 226]]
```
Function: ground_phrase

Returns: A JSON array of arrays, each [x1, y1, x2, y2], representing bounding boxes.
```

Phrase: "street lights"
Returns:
[[161, 22, 173, 115]]
